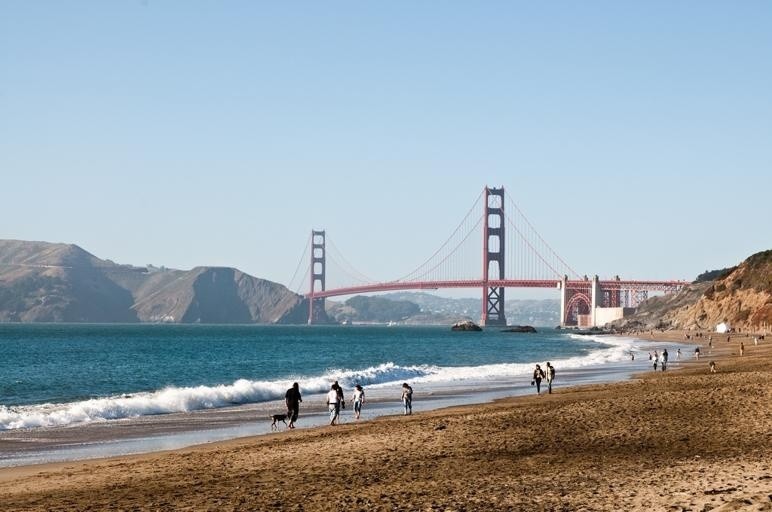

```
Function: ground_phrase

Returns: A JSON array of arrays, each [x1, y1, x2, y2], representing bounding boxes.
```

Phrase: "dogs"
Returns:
[[269, 412, 288, 429]]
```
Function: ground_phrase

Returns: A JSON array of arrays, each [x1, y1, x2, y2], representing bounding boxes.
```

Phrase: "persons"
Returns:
[[695, 346, 700, 360], [708, 359, 717, 373], [400, 383, 413, 416], [620, 328, 664, 335], [532, 364, 545, 395], [685, 327, 712, 346], [350, 384, 365, 419], [727, 327, 764, 356], [544, 361, 555, 394], [284, 382, 303, 429], [326, 384, 341, 425], [331, 381, 344, 419], [648, 348, 683, 372], [631, 354, 634, 360]]
